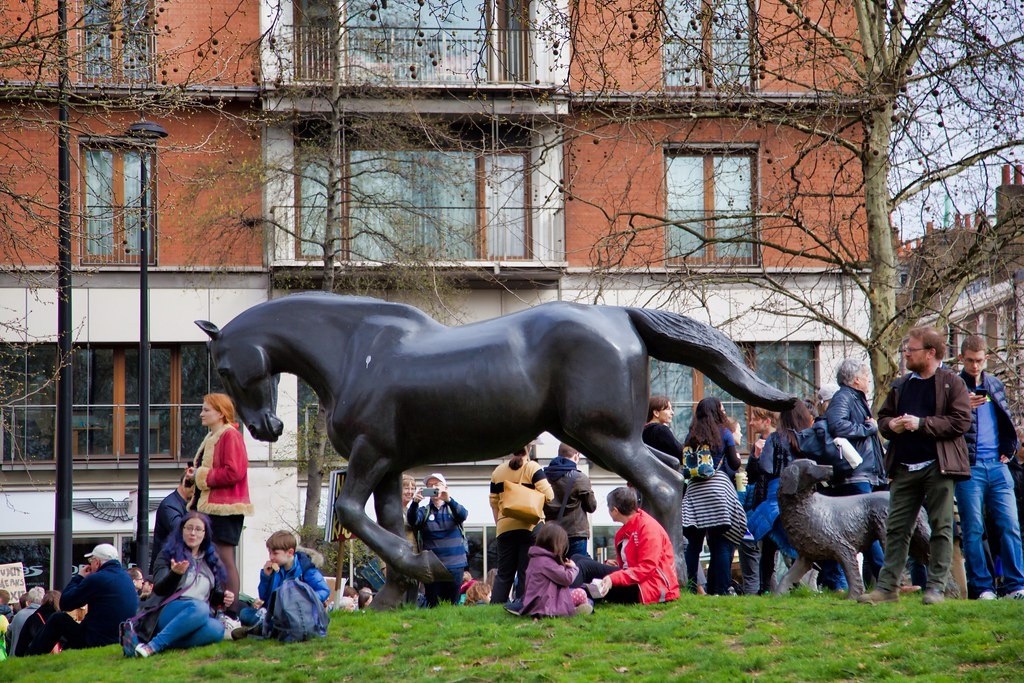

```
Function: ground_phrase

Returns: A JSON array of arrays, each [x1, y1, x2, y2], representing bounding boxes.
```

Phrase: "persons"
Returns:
[[0, 393, 373, 658], [401, 326, 1024, 615]]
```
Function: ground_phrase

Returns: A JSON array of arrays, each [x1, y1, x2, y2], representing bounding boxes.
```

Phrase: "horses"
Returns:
[[194, 295, 797, 613]]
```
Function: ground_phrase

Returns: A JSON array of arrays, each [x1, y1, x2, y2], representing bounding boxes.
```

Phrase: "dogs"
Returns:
[[774, 459, 963, 601]]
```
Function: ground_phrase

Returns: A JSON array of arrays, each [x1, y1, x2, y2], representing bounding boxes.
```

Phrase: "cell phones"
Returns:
[[421, 488, 438, 498], [976, 390, 988, 405]]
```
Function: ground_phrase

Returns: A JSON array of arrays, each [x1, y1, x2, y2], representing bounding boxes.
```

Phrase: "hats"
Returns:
[[84, 544, 118, 559], [818, 384, 840, 405], [423, 473, 447, 486], [144, 575, 154, 583]]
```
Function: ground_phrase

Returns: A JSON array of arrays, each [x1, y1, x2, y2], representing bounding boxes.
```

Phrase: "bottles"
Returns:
[[216, 610, 226, 628]]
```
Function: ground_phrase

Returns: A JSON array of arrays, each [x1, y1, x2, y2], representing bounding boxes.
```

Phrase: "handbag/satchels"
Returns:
[[502, 461, 546, 525], [120, 618, 138, 657]]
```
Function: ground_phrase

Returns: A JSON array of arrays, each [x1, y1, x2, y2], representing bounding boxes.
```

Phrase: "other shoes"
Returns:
[[503, 602, 524, 615], [231, 625, 247, 640], [574, 603, 594, 616]]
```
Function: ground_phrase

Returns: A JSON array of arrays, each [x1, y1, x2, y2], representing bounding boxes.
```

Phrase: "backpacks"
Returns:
[[787, 388, 868, 480], [682, 443, 725, 480], [263, 577, 330, 643]]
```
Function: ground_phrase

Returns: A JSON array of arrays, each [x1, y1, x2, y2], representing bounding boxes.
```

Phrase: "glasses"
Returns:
[[427, 481, 444, 487], [87, 558, 95, 563], [905, 347, 930, 353], [183, 525, 206, 534], [134, 578, 143, 582]]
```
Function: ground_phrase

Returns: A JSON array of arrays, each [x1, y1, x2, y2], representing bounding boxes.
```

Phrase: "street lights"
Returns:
[[121, 121, 168, 574]]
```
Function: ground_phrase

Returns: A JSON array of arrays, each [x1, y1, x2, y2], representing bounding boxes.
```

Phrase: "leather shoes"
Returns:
[[900, 585, 922, 593]]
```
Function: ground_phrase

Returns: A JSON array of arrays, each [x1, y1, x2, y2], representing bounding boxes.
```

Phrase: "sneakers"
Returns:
[[977, 591, 998, 601], [225, 615, 241, 630], [1006, 589, 1024, 599], [922, 588, 944, 605], [857, 589, 899, 605], [135, 643, 154, 658]]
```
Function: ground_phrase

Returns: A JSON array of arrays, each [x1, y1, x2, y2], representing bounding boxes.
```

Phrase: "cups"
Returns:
[[736, 474, 746, 492]]
